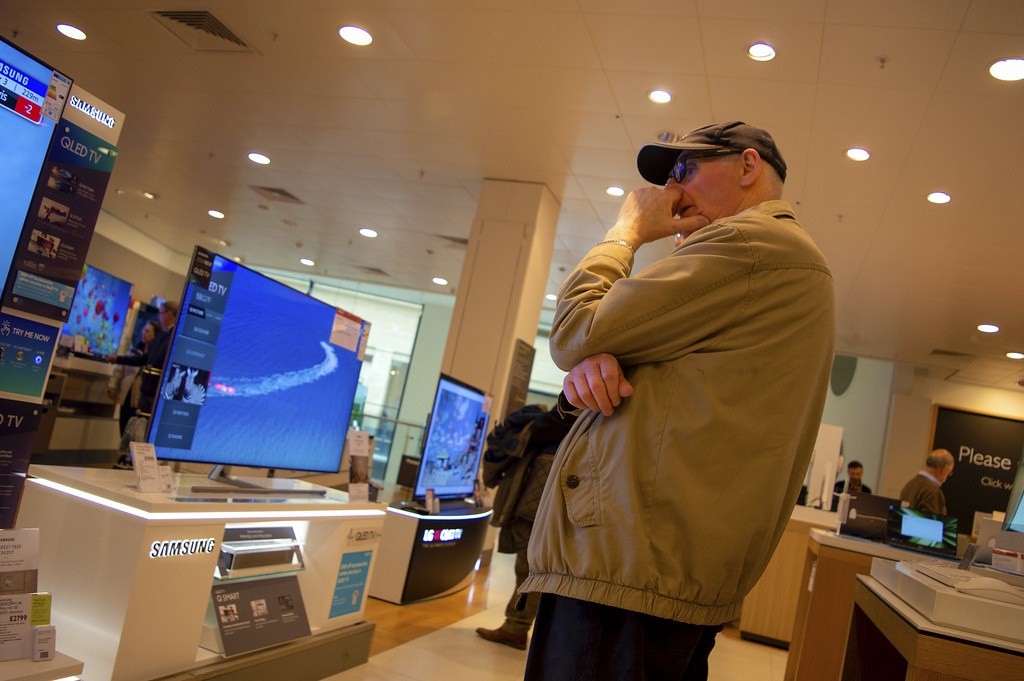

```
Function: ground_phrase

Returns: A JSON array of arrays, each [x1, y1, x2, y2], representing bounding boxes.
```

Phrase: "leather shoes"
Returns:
[[477, 627, 527, 650]]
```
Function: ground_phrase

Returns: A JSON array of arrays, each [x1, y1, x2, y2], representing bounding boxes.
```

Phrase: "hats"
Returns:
[[637, 121, 787, 186]]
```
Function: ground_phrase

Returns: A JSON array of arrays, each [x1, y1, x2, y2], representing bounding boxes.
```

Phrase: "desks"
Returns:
[[842, 573, 1024, 680]]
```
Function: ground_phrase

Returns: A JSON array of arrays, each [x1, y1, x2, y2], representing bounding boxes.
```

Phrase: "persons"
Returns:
[[475, 403, 579, 651], [516, 120, 838, 681], [830, 459, 871, 512], [900, 449, 954, 516], [104, 300, 179, 469]]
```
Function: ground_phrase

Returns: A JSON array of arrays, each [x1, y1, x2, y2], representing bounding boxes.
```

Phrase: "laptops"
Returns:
[[887, 506, 962, 561], [832, 491, 902, 542]]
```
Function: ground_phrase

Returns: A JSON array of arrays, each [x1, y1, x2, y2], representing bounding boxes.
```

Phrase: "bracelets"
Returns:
[[593, 239, 635, 254]]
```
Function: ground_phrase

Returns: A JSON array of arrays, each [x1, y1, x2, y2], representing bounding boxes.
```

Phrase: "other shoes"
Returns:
[[112, 456, 134, 470]]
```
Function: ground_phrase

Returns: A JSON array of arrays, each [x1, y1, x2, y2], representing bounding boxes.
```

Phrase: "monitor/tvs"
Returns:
[[0, 38, 373, 529], [412, 372, 493, 511]]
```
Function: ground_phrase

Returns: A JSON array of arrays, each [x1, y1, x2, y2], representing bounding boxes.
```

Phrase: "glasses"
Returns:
[[668, 149, 747, 182]]
